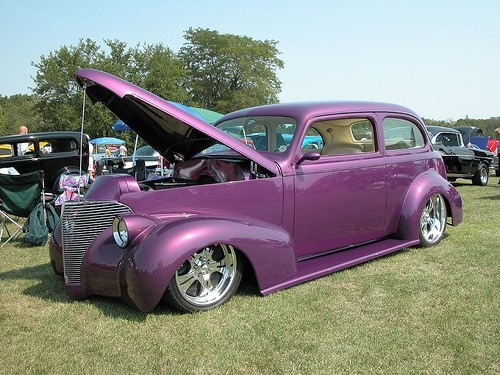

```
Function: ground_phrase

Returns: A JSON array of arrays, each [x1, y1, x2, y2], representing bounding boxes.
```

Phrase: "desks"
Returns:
[[103, 157, 121, 169], [124, 156, 158, 180]]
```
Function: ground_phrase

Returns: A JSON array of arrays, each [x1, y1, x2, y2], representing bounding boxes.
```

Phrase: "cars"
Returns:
[[47, 68, 464, 315], [381, 125, 493, 187], [0, 130, 324, 199]]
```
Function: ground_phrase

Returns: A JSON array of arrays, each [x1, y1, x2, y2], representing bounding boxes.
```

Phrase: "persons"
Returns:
[[11, 126, 28, 157], [85, 134, 93, 179], [105, 142, 127, 157], [493, 128, 500, 186]]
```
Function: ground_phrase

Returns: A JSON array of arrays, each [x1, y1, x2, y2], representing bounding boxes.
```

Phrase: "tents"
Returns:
[[191, 107, 247, 144], [90, 137, 125, 154]]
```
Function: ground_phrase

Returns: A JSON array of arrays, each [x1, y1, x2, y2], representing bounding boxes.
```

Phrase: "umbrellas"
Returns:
[[112, 120, 138, 167]]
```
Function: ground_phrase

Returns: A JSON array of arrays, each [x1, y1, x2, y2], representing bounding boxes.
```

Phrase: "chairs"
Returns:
[[104, 160, 146, 180], [0, 170, 45, 247], [320, 144, 363, 155], [55, 174, 88, 206]]
